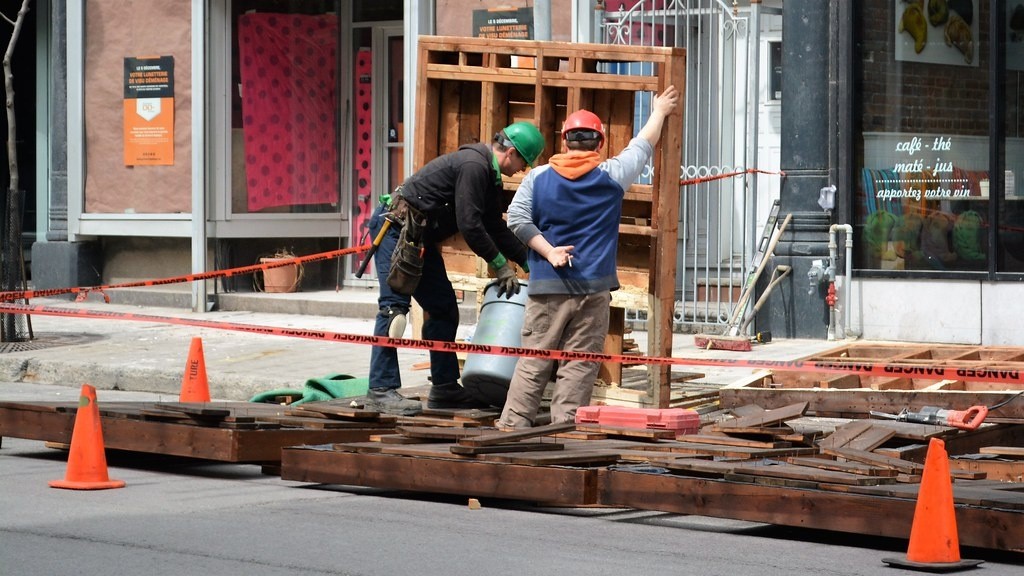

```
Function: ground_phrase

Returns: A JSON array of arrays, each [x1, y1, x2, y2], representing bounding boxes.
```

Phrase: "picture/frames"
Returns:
[[885, 0, 1024, 76]]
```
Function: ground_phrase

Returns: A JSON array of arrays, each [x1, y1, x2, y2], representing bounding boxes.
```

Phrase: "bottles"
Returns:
[[1005, 170, 1015, 198]]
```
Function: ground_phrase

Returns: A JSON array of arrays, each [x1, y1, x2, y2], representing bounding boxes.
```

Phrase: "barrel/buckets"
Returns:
[[461, 278, 529, 409]]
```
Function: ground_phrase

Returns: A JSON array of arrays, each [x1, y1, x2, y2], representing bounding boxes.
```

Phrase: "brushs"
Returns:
[[693, 214, 793, 351]]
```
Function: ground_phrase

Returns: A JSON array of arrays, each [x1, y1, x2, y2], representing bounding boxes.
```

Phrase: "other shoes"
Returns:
[[493, 418, 501, 426]]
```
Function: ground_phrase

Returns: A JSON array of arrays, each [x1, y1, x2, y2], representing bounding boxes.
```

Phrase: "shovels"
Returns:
[[736, 265, 792, 341]]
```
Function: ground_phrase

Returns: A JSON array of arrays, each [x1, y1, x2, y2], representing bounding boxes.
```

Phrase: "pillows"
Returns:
[[906, 167, 942, 220], [861, 166, 903, 223], [950, 165, 991, 198]]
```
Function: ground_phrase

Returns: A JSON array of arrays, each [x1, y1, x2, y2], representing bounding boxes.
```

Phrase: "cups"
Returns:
[[980, 181, 989, 197]]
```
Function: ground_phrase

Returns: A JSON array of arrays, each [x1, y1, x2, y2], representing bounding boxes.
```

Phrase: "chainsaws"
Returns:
[[869, 405, 989, 431]]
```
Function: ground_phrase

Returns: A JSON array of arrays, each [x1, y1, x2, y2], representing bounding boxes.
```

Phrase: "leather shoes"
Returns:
[[427, 385, 492, 410], [363, 388, 423, 417]]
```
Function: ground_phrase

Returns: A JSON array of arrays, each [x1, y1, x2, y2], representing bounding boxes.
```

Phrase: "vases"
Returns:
[[260, 255, 300, 293]]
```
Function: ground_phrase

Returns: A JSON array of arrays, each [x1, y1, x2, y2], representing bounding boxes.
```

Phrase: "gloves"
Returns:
[[495, 262, 521, 300]]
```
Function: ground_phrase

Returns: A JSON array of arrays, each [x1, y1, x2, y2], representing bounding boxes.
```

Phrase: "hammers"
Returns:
[[355, 212, 406, 279]]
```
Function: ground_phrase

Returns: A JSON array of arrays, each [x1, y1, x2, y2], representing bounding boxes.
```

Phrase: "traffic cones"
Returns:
[[49, 383, 125, 489], [178, 336, 209, 401], [882, 437, 986, 571]]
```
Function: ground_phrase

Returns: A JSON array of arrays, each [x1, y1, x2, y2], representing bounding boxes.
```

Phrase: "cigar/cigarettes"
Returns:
[[568, 256, 572, 267]]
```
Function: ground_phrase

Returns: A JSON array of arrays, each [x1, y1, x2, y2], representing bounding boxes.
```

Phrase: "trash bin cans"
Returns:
[[460, 279, 529, 408]]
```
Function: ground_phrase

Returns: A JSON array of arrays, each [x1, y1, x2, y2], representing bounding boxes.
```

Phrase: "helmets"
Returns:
[[504, 121, 545, 169], [561, 109, 606, 149]]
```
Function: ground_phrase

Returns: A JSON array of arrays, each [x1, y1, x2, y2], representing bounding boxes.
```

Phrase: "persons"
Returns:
[[496, 86, 678, 431], [364, 121, 545, 416]]
